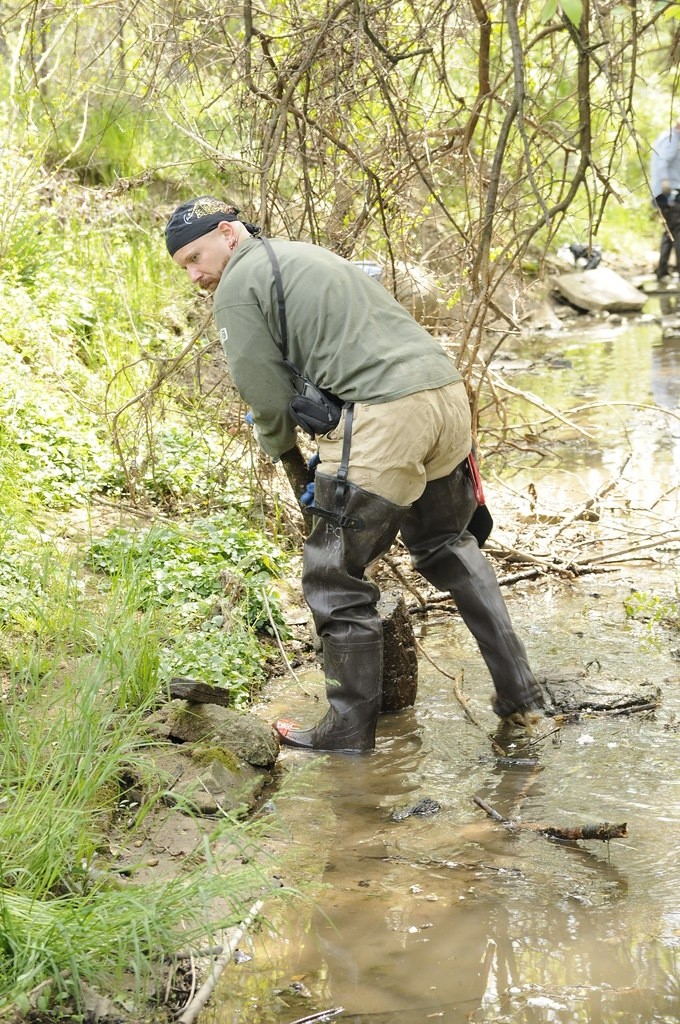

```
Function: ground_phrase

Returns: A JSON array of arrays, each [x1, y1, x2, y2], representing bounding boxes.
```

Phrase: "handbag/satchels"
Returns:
[[283, 360, 340, 439]]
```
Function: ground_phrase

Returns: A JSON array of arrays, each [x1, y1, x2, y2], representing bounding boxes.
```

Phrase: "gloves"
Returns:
[[300, 454, 319, 507]]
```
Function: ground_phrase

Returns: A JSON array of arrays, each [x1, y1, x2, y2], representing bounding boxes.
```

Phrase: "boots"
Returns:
[[272, 471, 413, 754], [400, 454, 544, 717]]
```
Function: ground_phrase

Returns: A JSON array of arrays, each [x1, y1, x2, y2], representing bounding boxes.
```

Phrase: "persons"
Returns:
[[165, 195, 550, 756], [649, 118, 680, 283]]
